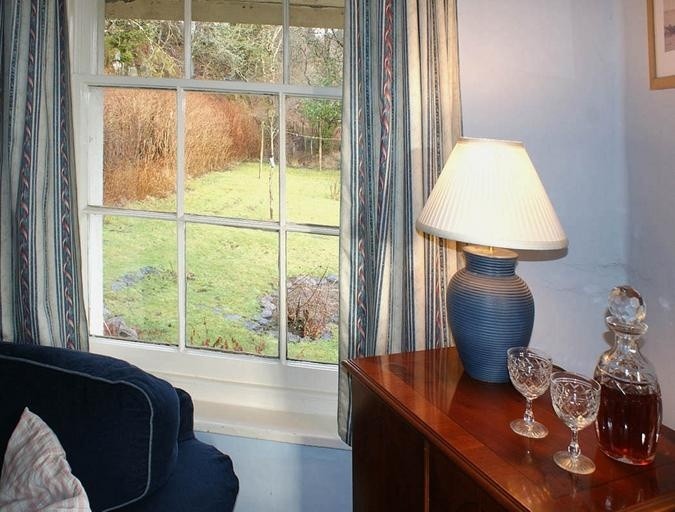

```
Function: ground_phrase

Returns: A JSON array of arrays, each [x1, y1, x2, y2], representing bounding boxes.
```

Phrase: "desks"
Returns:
[[343, 345, 674, 512]]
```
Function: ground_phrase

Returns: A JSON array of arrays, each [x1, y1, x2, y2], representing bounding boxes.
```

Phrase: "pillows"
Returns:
[[0, 406, 92, 512]]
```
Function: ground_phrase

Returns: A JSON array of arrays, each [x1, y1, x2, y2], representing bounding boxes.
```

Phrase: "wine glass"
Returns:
[[559, 476, 595, 511], [507, 437, 552, 512], [549, 371, 601, 475], [505, 345, 552, 439]]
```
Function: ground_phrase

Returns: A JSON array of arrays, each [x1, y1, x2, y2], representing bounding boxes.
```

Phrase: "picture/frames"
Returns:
[[645, 0, 674, 91]]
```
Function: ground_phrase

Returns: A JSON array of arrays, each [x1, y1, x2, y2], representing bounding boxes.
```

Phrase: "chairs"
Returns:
[[0, 342, 238, 512]]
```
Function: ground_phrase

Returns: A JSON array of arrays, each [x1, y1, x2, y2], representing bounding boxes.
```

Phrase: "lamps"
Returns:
[[416, 137, 569, 384]]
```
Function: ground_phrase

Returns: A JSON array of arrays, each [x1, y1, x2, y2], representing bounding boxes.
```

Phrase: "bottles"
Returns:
[[589, 444, 661, 512], [591, 285, 665, 467]]
